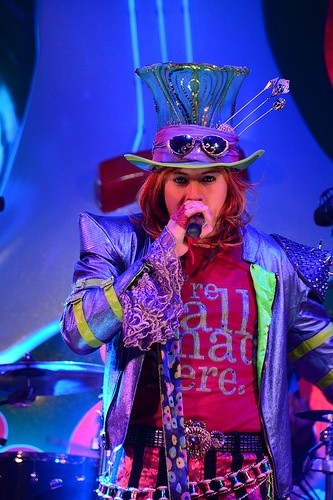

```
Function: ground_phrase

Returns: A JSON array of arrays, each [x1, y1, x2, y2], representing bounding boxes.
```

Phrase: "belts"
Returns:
[[122, 415, 267, 460]]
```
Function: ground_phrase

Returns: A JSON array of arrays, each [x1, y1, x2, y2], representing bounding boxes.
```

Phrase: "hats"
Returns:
[[121, 61, 289, 172]]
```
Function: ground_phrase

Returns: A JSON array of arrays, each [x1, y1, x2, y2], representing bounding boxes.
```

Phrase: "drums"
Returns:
[[0, 450, 99, 500]]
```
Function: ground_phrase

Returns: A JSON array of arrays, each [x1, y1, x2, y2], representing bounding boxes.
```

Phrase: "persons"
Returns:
[[58, 61, 333, 500]]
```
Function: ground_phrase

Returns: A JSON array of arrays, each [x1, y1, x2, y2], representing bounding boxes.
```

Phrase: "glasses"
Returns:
[[151, 133, 240, 160]]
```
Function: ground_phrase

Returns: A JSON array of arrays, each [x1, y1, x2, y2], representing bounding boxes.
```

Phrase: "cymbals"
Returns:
[[0, 361, 105, 399], [293, 410, 333, 423]]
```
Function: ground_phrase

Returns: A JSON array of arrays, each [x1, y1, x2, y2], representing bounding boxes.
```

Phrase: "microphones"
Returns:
[[186, 213, 204, 238]]
